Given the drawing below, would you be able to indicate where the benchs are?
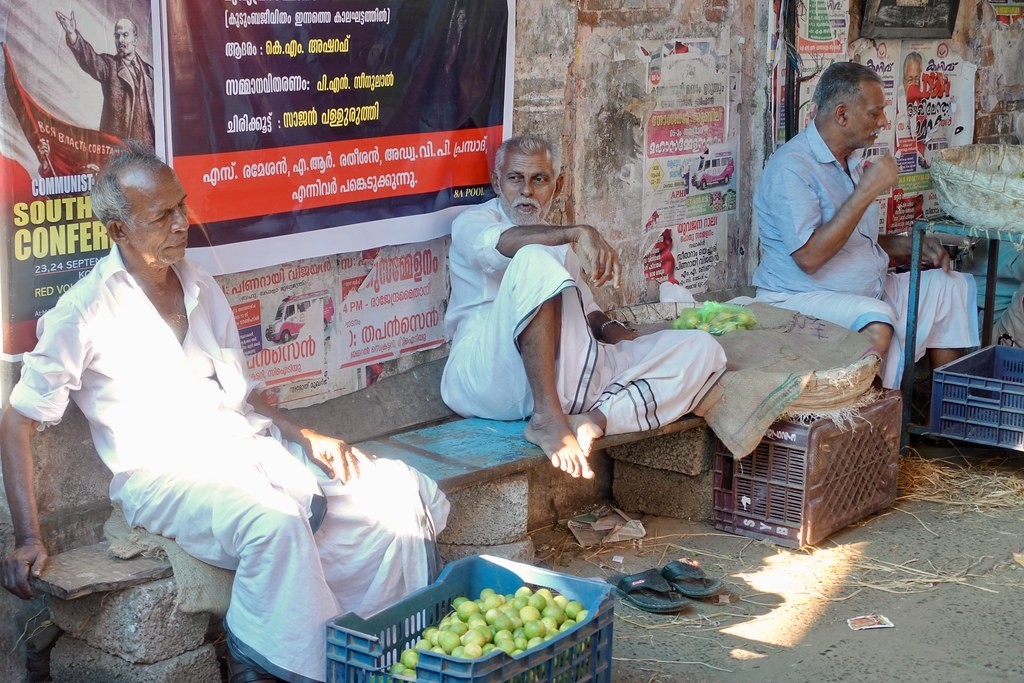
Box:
[21,417,713,683]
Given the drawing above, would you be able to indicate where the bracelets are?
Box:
[601,319,627,336]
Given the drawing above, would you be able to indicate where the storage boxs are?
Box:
[711,395,903,549]
[929,343,1024,452]
[325,554,618,683]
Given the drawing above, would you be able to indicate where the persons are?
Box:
[896,51,941,144]
[0,139,450,683]
[440,135,727,479]
[746,62,982,447]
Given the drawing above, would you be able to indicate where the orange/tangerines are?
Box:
[368,587,591,683]
[673,304,757,335]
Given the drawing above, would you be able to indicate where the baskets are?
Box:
[324,554,613,683]
[713,389,903,552]
[927,343,1024,453]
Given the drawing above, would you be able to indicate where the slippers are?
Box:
[661,561,725,599]
[604,568,688,613]
